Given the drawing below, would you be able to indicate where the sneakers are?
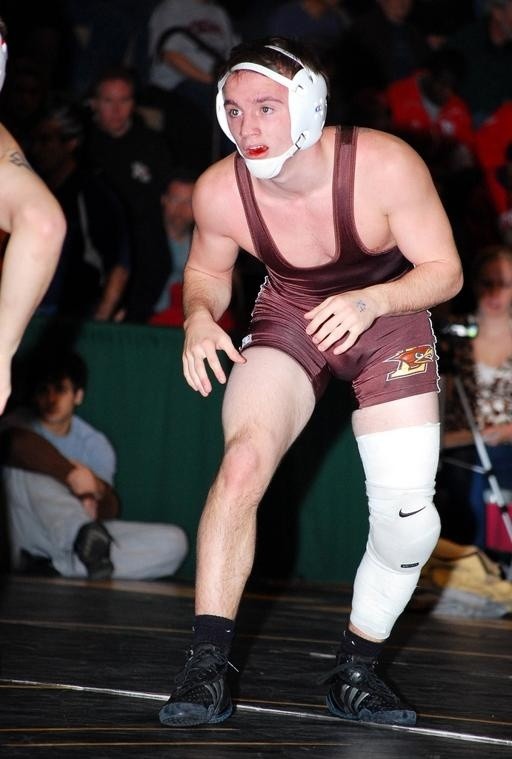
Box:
[74,521,120,580]
[160,642,240,726]
[317,649,417,725]
